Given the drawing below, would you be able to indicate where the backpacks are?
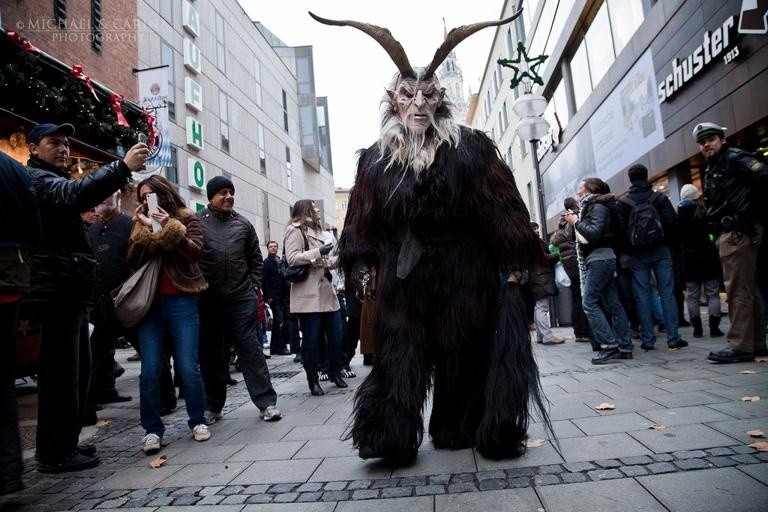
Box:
[627,203,667,249]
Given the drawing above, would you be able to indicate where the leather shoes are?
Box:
[263,347,292,359]
[35,441,101,473]
[306,374,324,396]
[590,350,633,366]
[754,346,768,356]
[707,345,754,362]
[327,374,349,388]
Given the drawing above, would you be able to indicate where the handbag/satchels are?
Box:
[108,259,165,330]
[278,257,310,283]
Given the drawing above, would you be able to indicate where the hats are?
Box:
[24,120,78,145]
[206,176,237,200]
[679,184,702,201]
[691,120,725,143]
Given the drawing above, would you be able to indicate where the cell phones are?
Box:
[138,134,146,148]
[146,193,162,232]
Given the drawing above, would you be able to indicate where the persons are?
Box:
[125,175,212,452]
[260,200,360,395]
[18,122,151,474]
[692,122,768,363]
[529,164,725,364]
[0,146,47,497]
[336,68,554,459]
[79,160,245,405]
[185,175,283,426]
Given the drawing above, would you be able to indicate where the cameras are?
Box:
[567,209,574,216]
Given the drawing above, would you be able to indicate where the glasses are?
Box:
[139,187,158,206]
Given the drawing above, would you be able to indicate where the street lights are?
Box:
[497,41,561,329]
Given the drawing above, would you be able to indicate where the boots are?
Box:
[690,315,705,338]
[709,315,724,338]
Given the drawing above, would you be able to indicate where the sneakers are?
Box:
[140,431,164,456]
[341,369,356,379]
[536,335,565,344]
[227,375,238,387]
[658,319,690,332]
[257,403,282,422]
[192,423,212,442]
[125,353,141,361]
[202,409,225,426]
[318,371,329,382]
[667,338,689,351]
[574,335,592,342]
[639,341,655,351]
[111,393,133,405]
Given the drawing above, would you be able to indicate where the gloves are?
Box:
[319,241,335,255]
